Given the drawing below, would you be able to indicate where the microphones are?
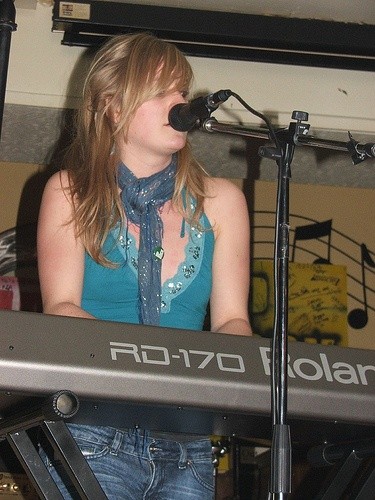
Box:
[168,90,231,132]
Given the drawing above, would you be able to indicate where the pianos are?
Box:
[0,309,375,425]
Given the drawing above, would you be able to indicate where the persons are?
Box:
[37,31,252,500]
